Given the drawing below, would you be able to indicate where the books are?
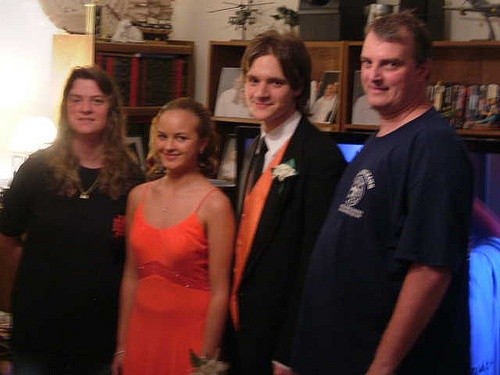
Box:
[98,56,189,107]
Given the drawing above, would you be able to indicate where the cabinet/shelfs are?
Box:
[205,40,500,214]
[52,35,195,174]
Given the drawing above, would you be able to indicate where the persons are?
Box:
[309,81,337,123]
[216,70,252,117]
[112,96,235,375]
[0,66,141,375]
[219,29,349,375]
[300,10,474,375]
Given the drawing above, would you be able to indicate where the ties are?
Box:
[251,135,269,188]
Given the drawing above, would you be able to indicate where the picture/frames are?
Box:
[310,70,341,124]
[211,65,259,120]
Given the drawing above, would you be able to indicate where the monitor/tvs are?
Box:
[235,126,376,190]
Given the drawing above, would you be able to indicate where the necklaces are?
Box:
[77,174,100,199]
